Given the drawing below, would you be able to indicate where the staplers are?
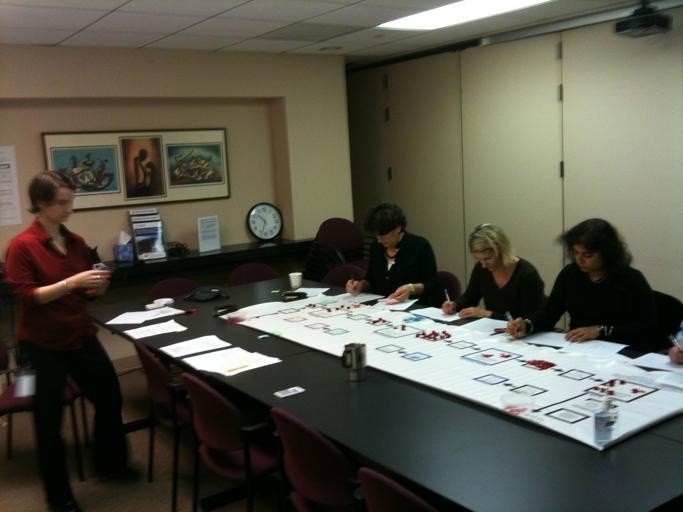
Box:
[282,292,307,302]
[214,304,238,317]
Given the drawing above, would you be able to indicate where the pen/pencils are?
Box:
[444,288,454,314]
[667,333,683,353]
[351,274,354,291]
[505,310,521,338]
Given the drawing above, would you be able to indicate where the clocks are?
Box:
[246,202,283,241]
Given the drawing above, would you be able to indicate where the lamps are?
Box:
[615,0,673,38]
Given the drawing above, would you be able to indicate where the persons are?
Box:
[668,319,683,364]
[3,170,140,512]
[505,218,661,351]
[442,223,546,319]
[345,202,439,301]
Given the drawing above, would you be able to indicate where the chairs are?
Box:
[228,263,281,288]
[1,339,83,472]
[84,272,683,512]
[322,265,367,289]
[307,218,362,269]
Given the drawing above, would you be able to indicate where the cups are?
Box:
[287,272,303,290]
[340,342,367,371]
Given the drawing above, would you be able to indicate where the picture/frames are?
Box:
[41,128,231,212]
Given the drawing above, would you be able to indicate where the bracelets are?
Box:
[598,319,617,340]
[409,283,416,296]
[525,318,534,335]
[62,278,71,295]
